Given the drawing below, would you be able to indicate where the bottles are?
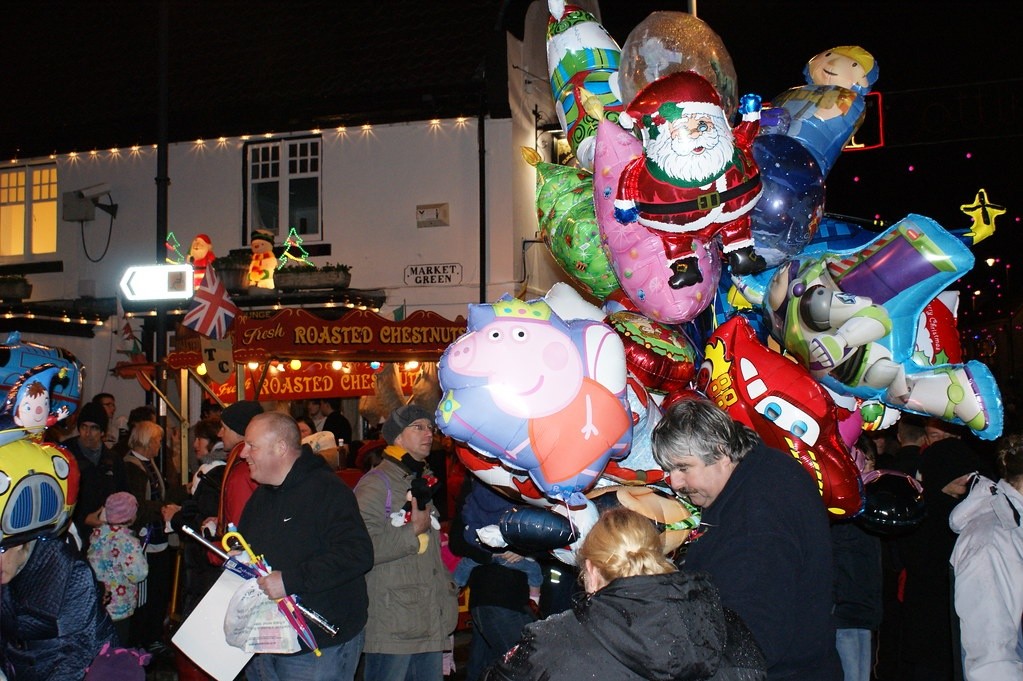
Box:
[336,439,348,470]
[228,522,242,548]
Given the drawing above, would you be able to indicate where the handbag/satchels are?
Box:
[84,642,152,681]
[244,596,302,653]
[224,577,265,651]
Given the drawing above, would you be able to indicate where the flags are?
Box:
[116,299,141,354]
[182,262,237,342]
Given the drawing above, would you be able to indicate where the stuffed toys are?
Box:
[388,475,441,556]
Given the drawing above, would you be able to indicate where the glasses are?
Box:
[406,425,433,432]
[103,402,115,407]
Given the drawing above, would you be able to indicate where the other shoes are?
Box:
[528,599,547,620]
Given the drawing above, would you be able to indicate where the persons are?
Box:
[87,492,149,653]
[827,413,1023,681]
[350,410,461,681]
[446,477,543,614]
[185,233,217,293]
[157,400,263,607]
[291,397,352,446]
[478,508,770,681]
[225,411,375,681]
[533,488,622,622]
[649,398,845,681]
[60,392,187,652]
[0,538,120,681]
[448,470,530,681]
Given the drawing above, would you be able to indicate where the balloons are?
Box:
[427,0,1006,552]
[12,367,70,445]
[0,425,81,546]
[0,330,84,426]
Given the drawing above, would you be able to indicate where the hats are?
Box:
[221,400,265,437]
[77,402,108,434]
[381,404,435,445]
[106,491,138,524]
[918,436,977,497]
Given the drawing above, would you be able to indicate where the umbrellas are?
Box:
[221,531,321,657]
